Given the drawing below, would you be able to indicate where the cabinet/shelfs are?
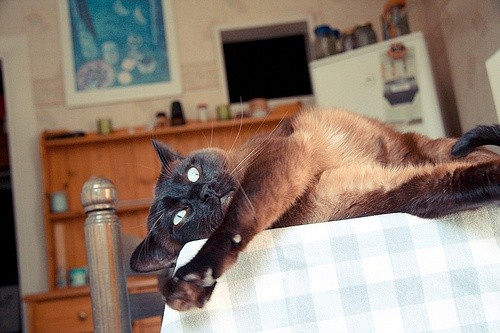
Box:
[23,101,302,333]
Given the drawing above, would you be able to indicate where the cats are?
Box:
[128,106,500,312]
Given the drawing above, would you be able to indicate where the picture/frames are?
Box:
[59,0,183,106]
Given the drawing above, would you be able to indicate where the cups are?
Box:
[217,105,228,120]
[97,119,111,135]
[70,268,88,288]
[51,190,67,213]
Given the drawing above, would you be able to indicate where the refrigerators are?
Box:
[308,31,446,139]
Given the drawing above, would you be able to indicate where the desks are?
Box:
[160,198,499,333]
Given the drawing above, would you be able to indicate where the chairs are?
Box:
[82,176,171,333]
